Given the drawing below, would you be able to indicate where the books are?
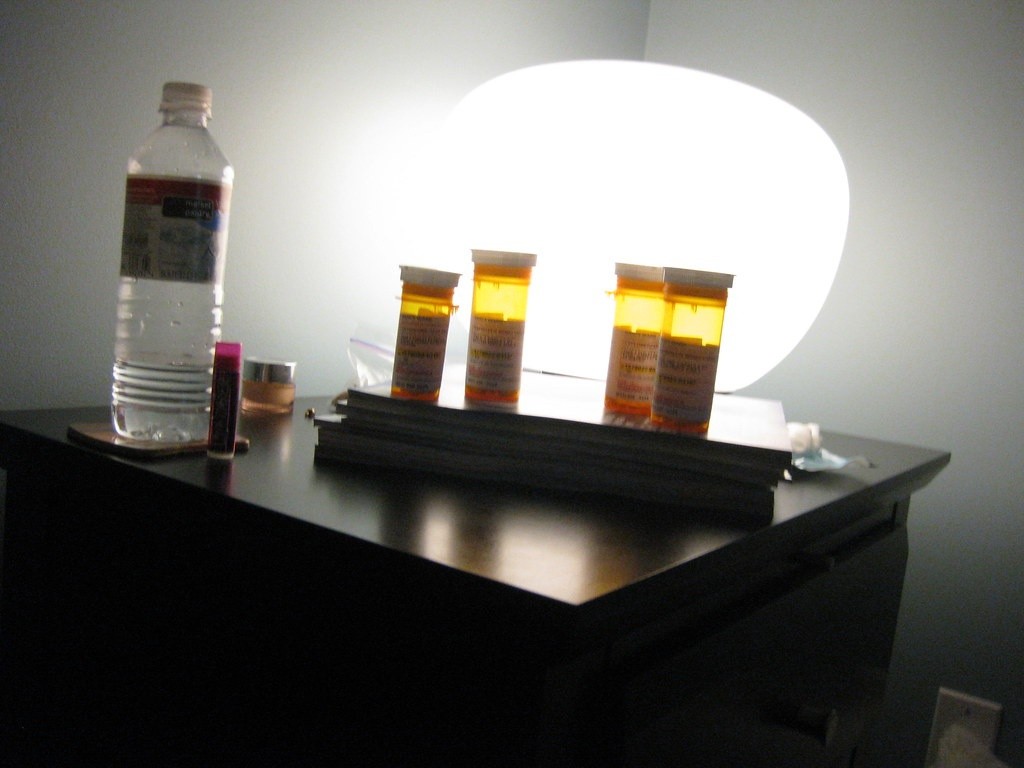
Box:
[312,362,792,516]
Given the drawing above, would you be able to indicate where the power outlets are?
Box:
[922,686,1002,768]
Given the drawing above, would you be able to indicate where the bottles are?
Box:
[393,266,462,400]
[112,82,234,440]
[605,263,666,416]
[652,267,737,432]
[465,249,538,408]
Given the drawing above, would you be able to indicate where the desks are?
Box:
[0,391,951,768]
